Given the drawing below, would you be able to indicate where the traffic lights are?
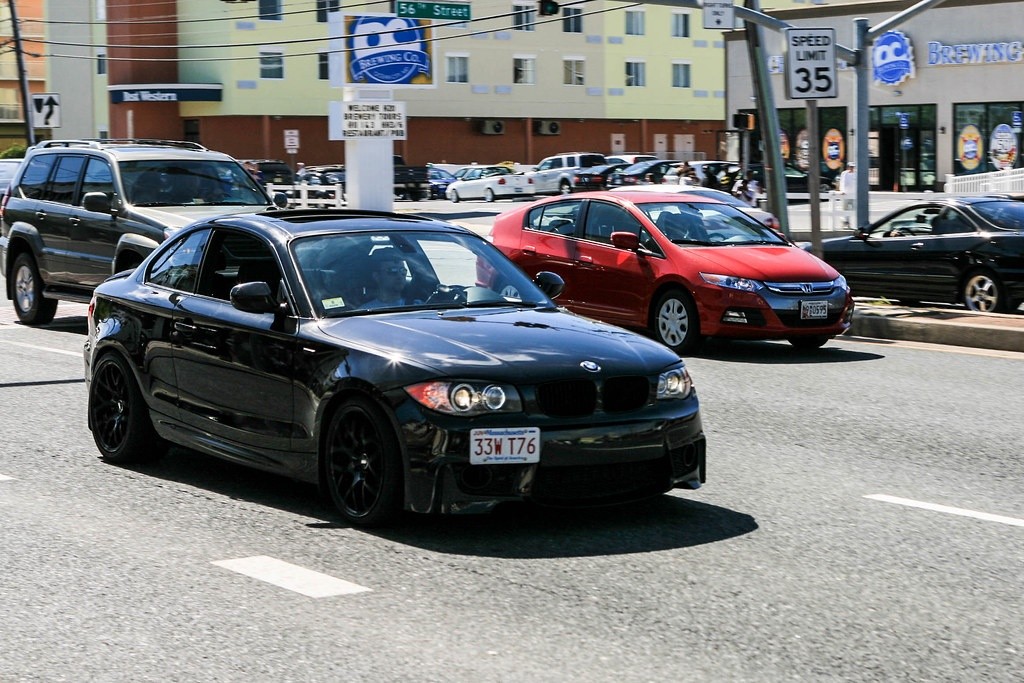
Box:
[537,0,559,17]
[732,113,755,130]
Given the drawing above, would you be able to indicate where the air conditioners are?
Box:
[538,121,561,134]
[480,120,506,135]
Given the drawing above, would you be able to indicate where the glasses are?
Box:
[379,267,407,276]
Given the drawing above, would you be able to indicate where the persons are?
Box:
[295,162,306,178]
[182,171,212,203]
[889,216,940,237]
[243,162,264,184]
[839,162,858,230]
[630,161,763,208]
[358,259,427,311]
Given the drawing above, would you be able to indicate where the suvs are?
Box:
[0,139,287,324]
[528,152,604,194]
[236,153,458,209]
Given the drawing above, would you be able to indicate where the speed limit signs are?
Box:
[784,26,838,99]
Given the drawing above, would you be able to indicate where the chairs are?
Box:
[938,220,966,232]
[657,209,672,236]
[593,205,621,237]
[237,242,282,296]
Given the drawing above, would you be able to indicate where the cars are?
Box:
[488,193,854,354]
[83,209,706,525]
[611,184,779,244]
[805,195,1024,312]
[456,167,474,177]
[445,167,535,203]
[575,155,832,193]
[0,158,26,194]
